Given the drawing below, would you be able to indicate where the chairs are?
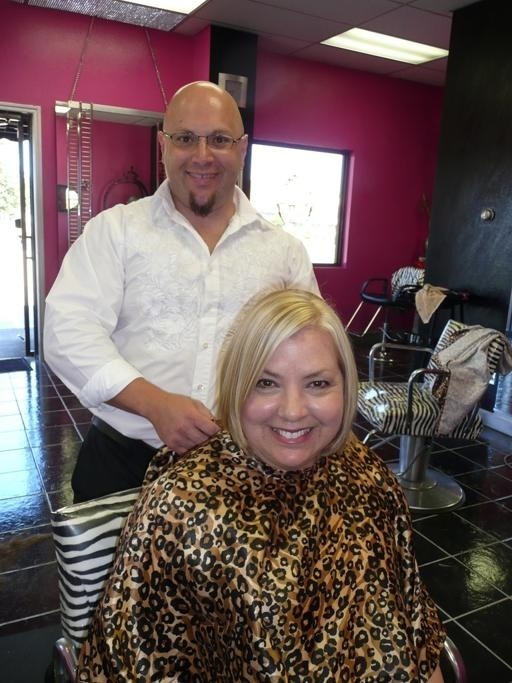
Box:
[354,320,505,512]
[50,485,466,683]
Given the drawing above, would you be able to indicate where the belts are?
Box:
[91,414,152,456]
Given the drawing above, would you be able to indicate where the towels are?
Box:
[415,284,449,324]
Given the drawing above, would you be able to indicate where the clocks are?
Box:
[218,72,248,109]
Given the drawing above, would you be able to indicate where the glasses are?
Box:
[163,133,243,150]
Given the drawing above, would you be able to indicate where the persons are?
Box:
[37,78,326,511]
[74,281,454,683]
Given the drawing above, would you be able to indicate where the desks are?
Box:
[406,289,467,348]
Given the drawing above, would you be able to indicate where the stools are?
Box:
[343,276,422,364]
